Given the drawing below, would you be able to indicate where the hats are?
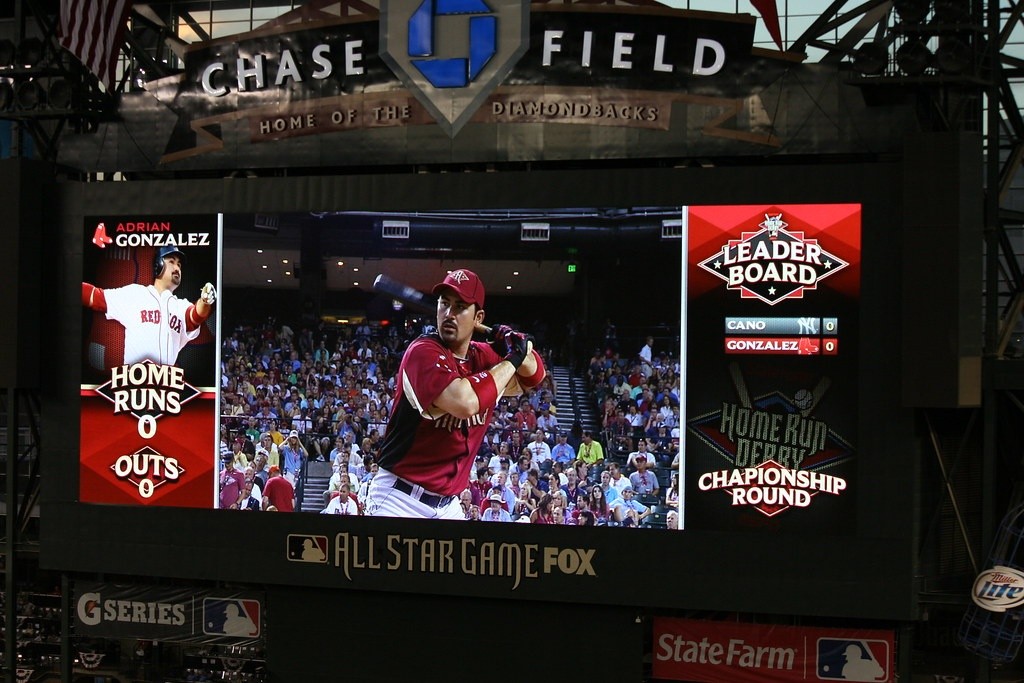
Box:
[221,451,235,462]
[431,269,485,309]
[267,465,280,479]
[258,449,270,456]
[152,244,186,268]
[636,455,648,462]
[559,430,568,437]
[475,456,484,463]
[540,403,550,411]
[498,458,510,464]
[231,437,244,446]
[289,430,300,439]
[487,494,507,504]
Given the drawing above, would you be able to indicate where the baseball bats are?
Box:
[371,273,498,337]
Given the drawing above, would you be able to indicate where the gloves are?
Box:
[484,324,536,370]
[200,282,217,306]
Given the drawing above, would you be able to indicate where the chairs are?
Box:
[219,323,680,530]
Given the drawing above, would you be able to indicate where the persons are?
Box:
[81,243,218,377]
[364,269,546,520]
[219,317,682,529]
